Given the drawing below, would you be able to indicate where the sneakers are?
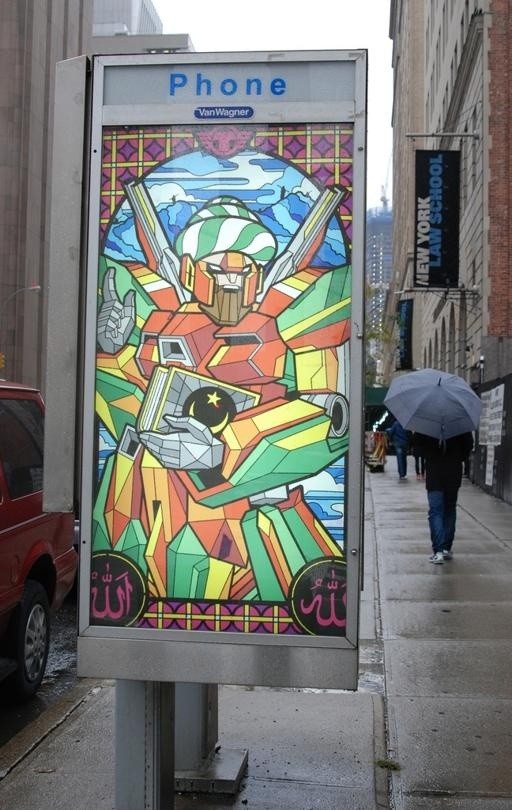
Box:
[400,475,408,480]
[429,550,453,564]
[416,475,426,480]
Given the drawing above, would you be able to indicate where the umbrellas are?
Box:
[382,366,486,446]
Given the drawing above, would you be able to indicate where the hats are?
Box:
[176,195,278,268]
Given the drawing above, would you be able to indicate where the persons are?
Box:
[384,425,393,455]
[410,430,427,479]
[389,419,413,480]
[417,432,474,565]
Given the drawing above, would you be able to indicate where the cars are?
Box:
[0,383,80,702]
